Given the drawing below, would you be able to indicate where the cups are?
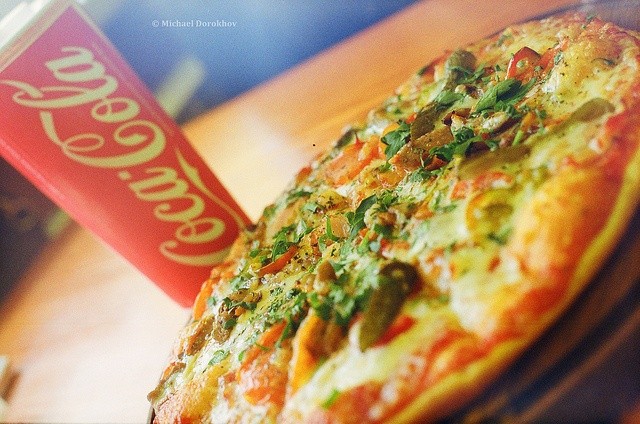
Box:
[1,0,256,314]
